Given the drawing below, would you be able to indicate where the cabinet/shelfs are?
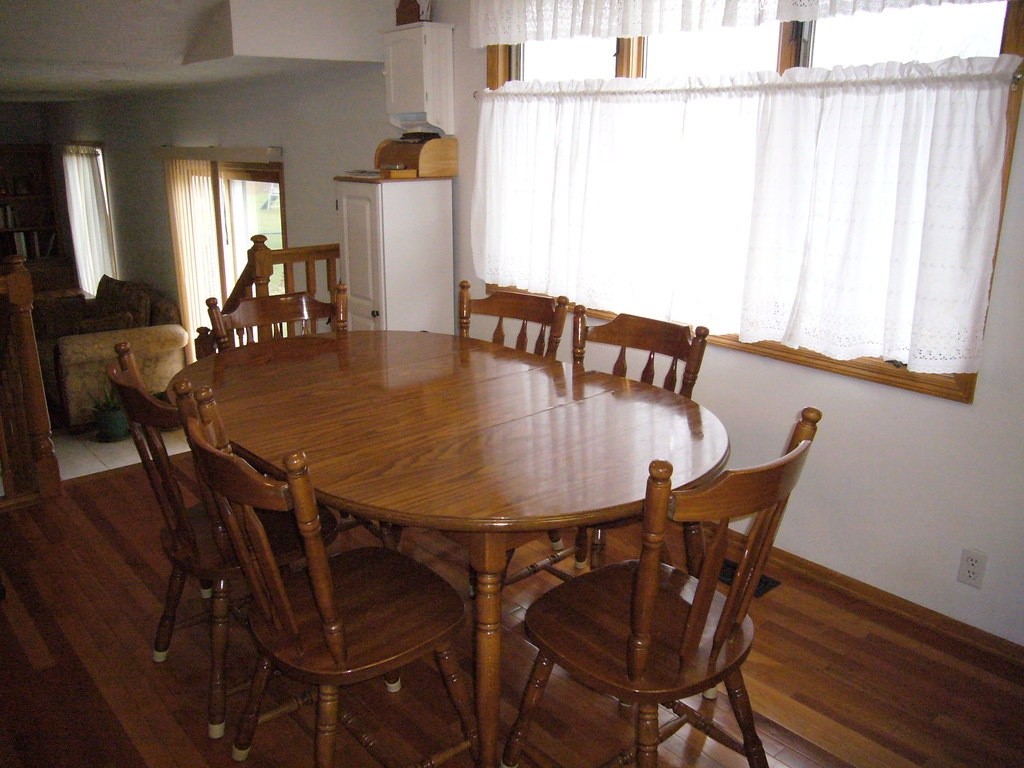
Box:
[0,144,76,289]
[382,22,455,136]
[334,172,453,334]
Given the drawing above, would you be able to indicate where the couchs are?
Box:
[32,273,189,434]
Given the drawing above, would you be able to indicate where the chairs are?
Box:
[501,407,823,768]
[184,385,480,768]
[105,342,340,740]
[206,282,401,553]
[501,305,710,590]
[391,281,569,599]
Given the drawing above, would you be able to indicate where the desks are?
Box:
[168,330,731,768]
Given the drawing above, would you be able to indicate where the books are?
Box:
[14,231,55,259]
[0,205,15,229]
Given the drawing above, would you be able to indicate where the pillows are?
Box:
[78,311,132,334]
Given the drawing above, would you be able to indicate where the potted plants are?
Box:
[87,384,130,442]
